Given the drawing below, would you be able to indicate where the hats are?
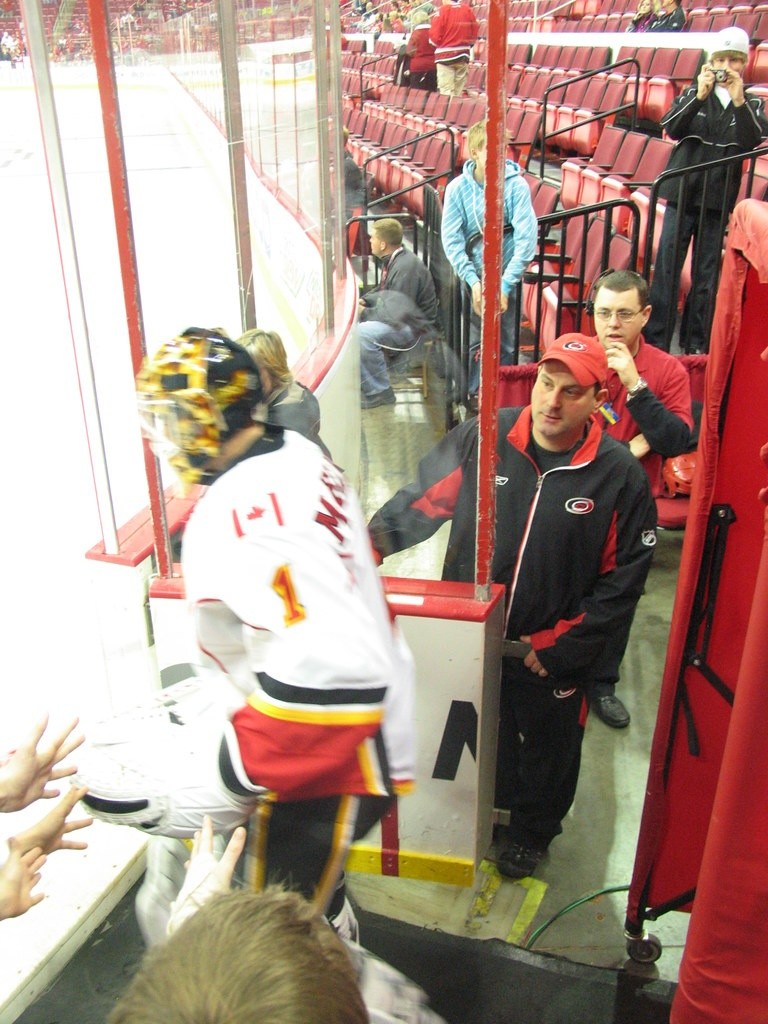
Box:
[708,26,749,56]
[536,333,610,398]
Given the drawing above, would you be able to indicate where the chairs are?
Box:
[0,0,312,63]
[325,0,768,529]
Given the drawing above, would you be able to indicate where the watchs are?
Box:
[629,377,647,395]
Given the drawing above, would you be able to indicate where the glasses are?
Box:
[594,305,648,323]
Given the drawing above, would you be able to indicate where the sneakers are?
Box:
[497,839,544,877]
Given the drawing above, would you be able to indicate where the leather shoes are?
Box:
[357,386,396,409]
[590,690,629,728]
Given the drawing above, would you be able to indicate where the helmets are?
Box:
[133,326,269,489]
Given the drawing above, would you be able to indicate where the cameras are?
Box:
[708,69,727,83]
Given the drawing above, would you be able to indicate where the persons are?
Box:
[643,0,686,33]
[440,122,538,398]
[0,326,415,1024]
[626,0,664,32]
[360,219,437,408]
[645,27,768,353]
[0,0,218,65]
[238,329,332,464]
[584,269,693,727]
[406,12,437,92]
[344,127,363,206]
[355,0,434,31]
[366,331,658,881]
[430,0,480,96]
